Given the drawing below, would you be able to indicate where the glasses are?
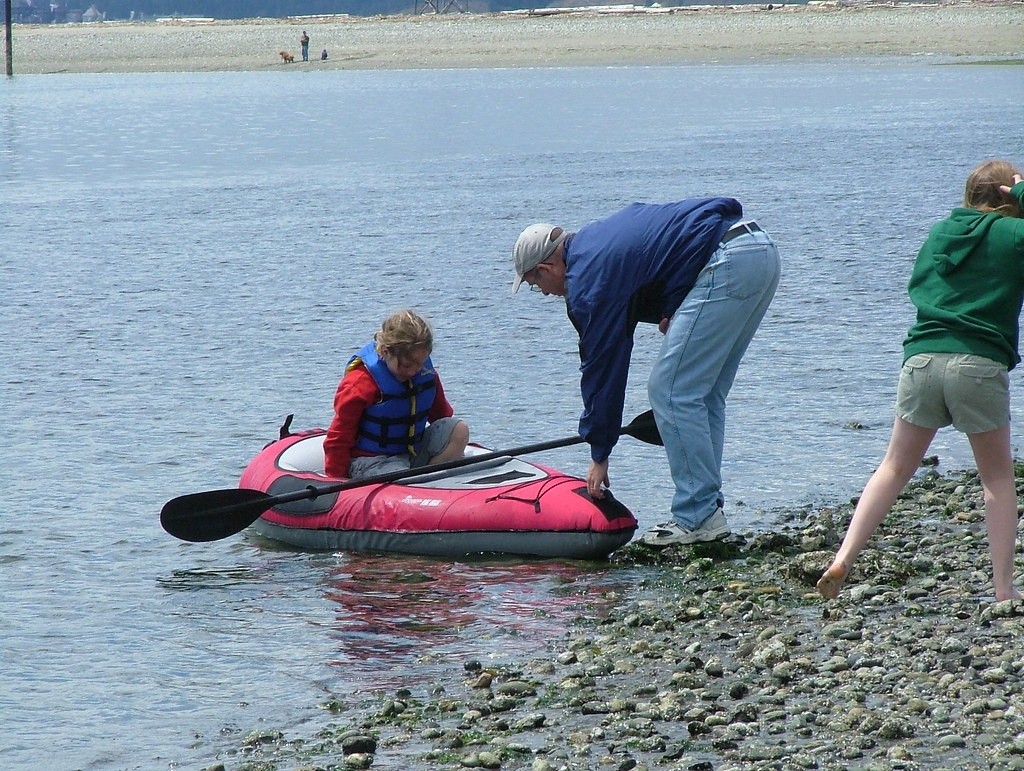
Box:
[531,270,542,292]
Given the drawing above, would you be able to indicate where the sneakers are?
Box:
[640,507,731,545]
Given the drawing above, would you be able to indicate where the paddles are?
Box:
[159,405,665,544]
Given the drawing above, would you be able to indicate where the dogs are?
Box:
[279,51,293,63]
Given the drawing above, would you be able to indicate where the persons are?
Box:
[300,31,309,61]
[322,310,469,480]
[511,199,781,545]
[815,160,1024,599]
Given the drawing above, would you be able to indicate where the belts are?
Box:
[714,221,761,250]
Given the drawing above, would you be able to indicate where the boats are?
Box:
[236,414,640,562]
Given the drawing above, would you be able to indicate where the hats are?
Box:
[512,223,567,294]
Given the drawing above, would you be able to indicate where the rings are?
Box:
[590,487,594,490]
